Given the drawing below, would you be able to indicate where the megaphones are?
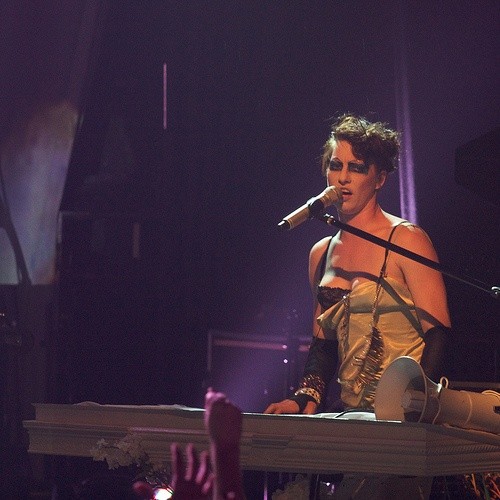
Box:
[374,355,500,437]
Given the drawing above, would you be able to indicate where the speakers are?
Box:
[207,330,290,413]
[52,210,186,404]
[289,335,343,413]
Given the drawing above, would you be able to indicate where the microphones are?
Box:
[277,185,342,232]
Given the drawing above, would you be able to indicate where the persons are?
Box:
[128,383,248,500]
[252,111,459,500]
